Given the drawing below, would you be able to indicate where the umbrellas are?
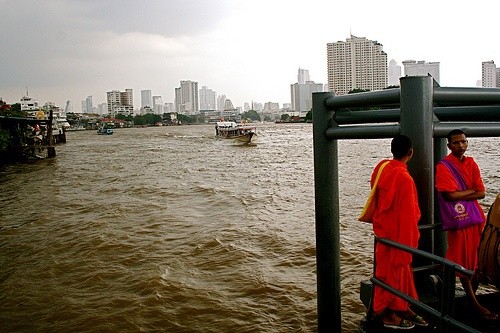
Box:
[0,104,12,109]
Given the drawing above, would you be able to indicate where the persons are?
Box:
[435,129,498,323]
[370,134,428,330]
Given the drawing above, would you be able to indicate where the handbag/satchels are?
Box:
[357,161,391,223]
[435,159,483,231]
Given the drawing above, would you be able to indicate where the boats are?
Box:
[20,88,86,144]
[215,117,258,147]
[97,127,114,135]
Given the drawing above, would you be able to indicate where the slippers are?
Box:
[406,314,430,326]
[384,317,415,329]
